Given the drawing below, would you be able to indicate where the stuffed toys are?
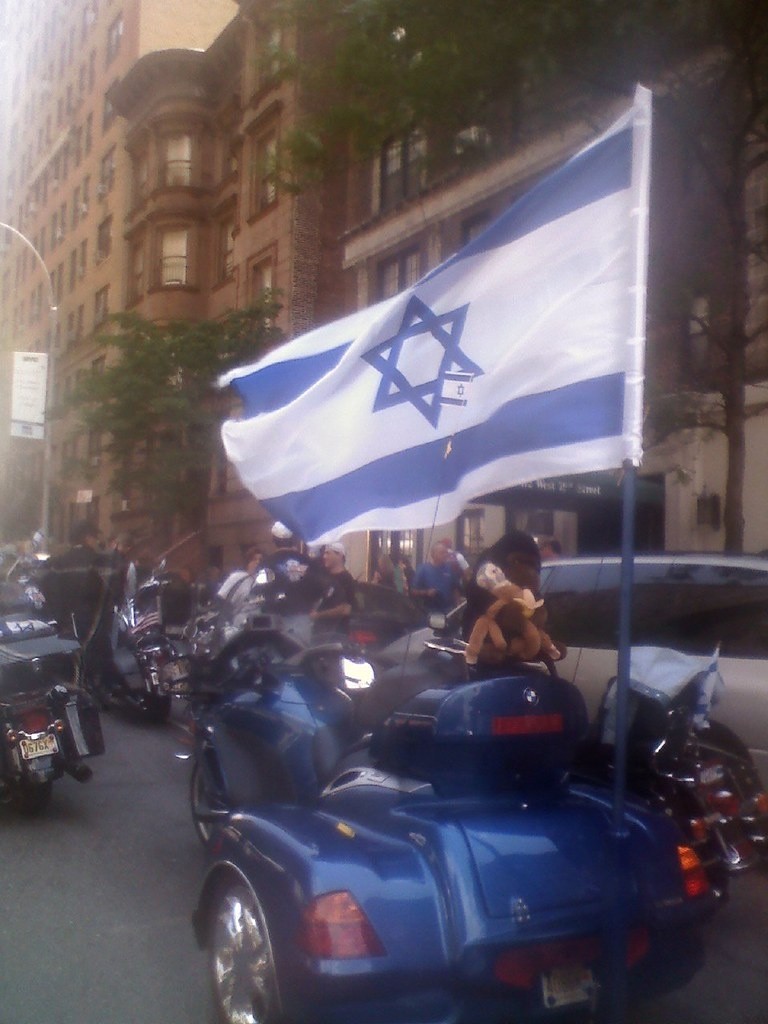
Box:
[461,529,568,678]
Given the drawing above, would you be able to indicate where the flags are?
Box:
[211,81,656,555]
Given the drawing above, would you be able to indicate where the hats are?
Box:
[271,521,293,545]
[326,542,346,557]
[69,518,103,536]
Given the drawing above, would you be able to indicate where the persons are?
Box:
[307,543,359,643]
[202,519,323,639]
[372,538,471,620]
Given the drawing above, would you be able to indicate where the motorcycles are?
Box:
[7,531,768,925]
[3,574,106,810]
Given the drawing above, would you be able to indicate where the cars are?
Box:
[367,553,766,772]
[173,642,730,1022]
